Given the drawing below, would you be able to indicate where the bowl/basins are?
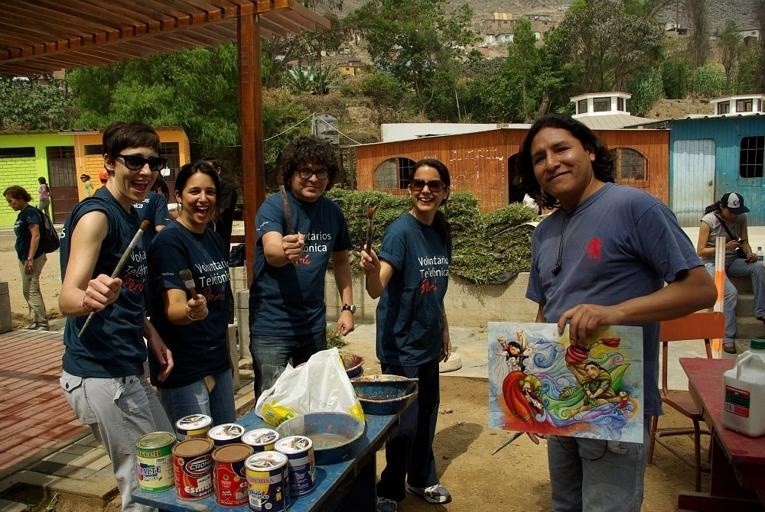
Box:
[275,411,364,466]
[348,374,418,416]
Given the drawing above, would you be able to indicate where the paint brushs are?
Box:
[76,220,147,338]
[365,206,379,257]
[277,173,295,237]
[492,432,526,456]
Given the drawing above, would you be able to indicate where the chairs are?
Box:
[648,308,728,493]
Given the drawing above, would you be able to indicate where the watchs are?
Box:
[338,304,358,314]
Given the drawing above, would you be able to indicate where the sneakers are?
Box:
[376,496,398,511]
[406,482,451,504]
[724,342,735,353]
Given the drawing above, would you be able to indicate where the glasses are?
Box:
[297,168,331,180]
[119,154,168,171]
[410,178,447,192]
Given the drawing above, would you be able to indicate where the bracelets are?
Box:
[23,255,32,259]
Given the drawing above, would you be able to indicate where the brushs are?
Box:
[179,269,200,301]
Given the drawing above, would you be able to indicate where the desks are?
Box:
[123,399,410,511]
[678,352,764,512]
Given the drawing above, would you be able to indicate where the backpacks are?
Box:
[37,207,60,253]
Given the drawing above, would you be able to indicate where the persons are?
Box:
[359,157,454,512]
[128,190,166,239]
[78,173,93,199]
[36,177,53,220]
[57,122,177,512]
[515,113,717,509]
[696,192,765,353]
[243,133,358,406]
[87,168,107,197]
[204,161,237,264]
[144,160,237,434]
[2,185,60,334]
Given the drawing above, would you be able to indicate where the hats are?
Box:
[721,192,750,214]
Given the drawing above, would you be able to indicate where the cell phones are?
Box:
[738,240,744,243]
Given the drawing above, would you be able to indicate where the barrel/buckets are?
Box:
[722,336,764,437]
